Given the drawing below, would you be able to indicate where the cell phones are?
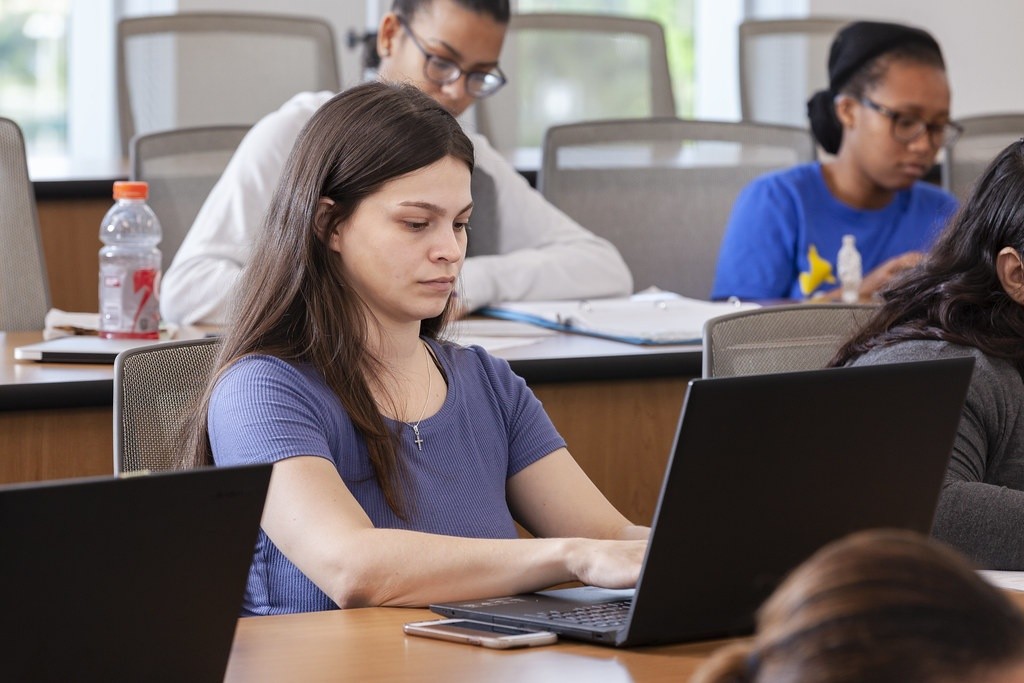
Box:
[403,618,557,650]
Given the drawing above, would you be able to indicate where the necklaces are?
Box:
[375,340,433,450]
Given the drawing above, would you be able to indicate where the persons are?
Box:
[708,19,962,308]
[166,81,651,623]
[824,140,1023,573]
[685,523,1023,682]
[158,1,632,325]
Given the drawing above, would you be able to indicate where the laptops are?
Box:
[428,355,979,651]
[0,461,276,683]
[14,336,166,363]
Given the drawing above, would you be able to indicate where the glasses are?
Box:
[854,89,964,149]
[394,16,507,99]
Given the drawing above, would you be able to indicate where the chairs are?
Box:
[0,0,1023,475]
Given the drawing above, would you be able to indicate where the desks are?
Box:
[222,556,1024,683]
[0,303,797,528]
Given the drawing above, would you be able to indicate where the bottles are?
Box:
[99,181,162,342]
[837,235,862,304]
